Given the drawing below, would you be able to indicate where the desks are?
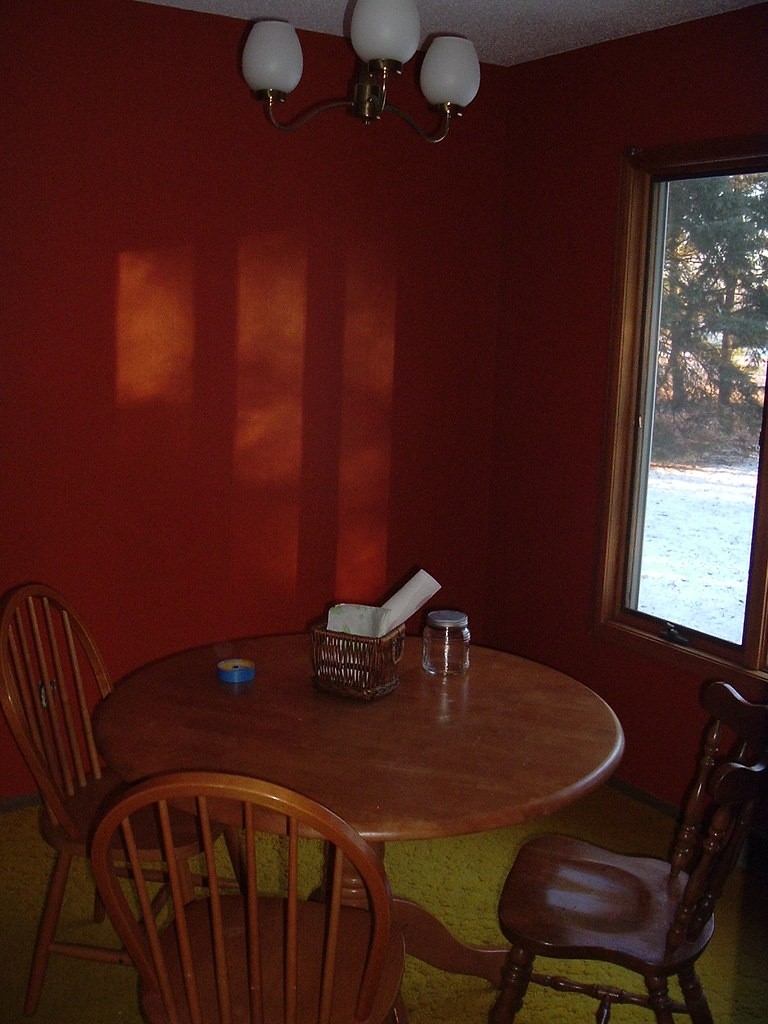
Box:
[94,633,624,1023]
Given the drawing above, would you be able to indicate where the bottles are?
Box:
[420,611,471,674]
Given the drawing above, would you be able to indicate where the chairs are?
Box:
[488,681,768,1024]
[91,771,406,1024]
[0,582,246,1016]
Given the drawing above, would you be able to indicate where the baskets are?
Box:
[310,621,407,700]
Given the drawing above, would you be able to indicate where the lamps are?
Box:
[242,1,481,146]
